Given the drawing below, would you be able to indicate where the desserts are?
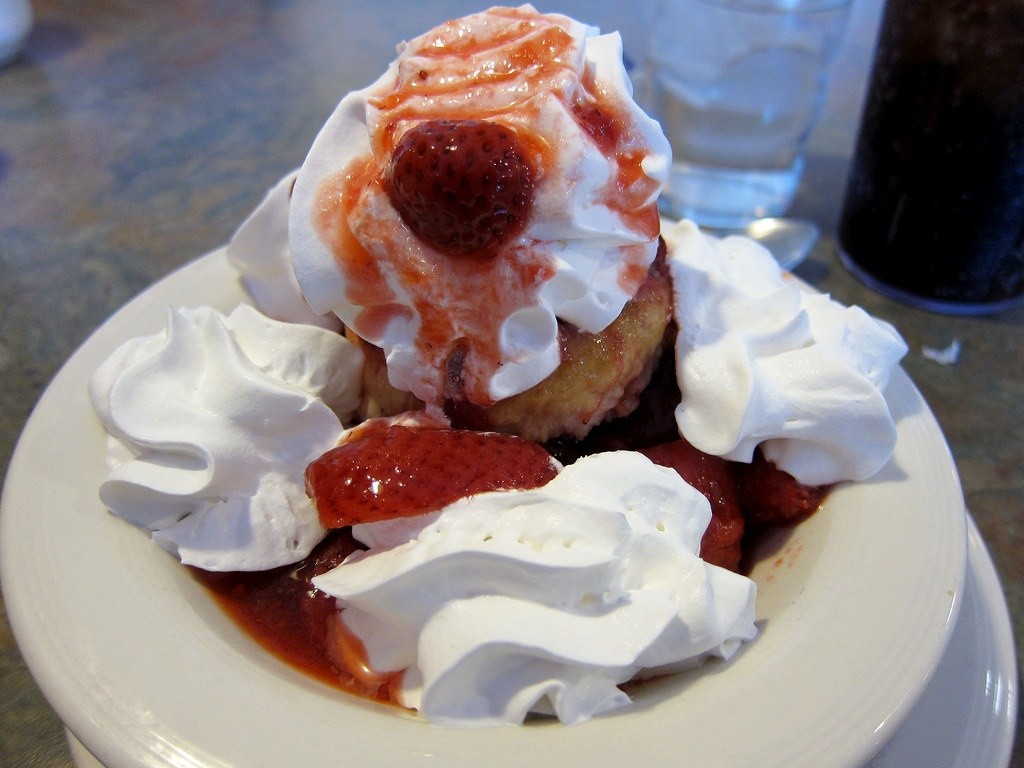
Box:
[86,0,915,728]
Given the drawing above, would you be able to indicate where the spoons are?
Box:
[743,218,821,272]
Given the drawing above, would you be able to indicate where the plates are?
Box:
[64,510,1017,767]
[1,243,967,767]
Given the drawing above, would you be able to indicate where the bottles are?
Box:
[835,0,1024,317]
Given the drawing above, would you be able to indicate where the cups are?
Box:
[629,1,854,231]
